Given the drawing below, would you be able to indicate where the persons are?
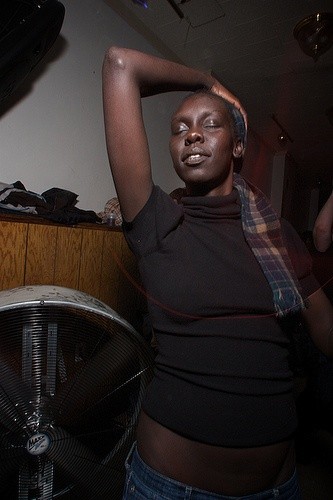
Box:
[312,190,333,255]
[102,45,332,500]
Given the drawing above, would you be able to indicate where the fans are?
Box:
[1,285,148,500]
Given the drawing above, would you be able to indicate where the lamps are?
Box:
[272,116,294,145]
[294,12,333,61]
